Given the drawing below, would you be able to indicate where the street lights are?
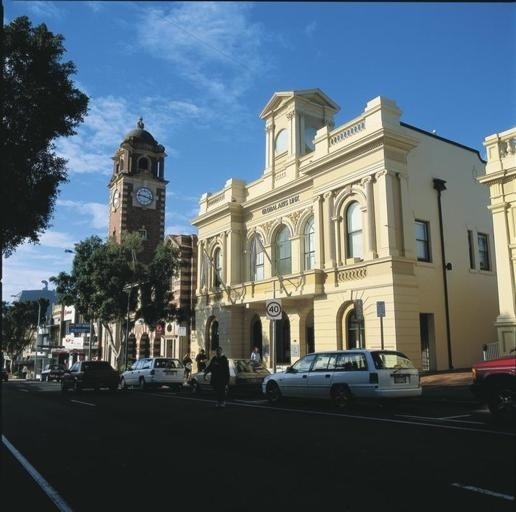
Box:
[64,248,76,254]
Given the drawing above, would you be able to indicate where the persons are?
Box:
[182,350,208,378]
[251,347,260,363]
[203,347,229,408]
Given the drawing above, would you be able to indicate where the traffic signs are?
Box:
[266,299,282,320]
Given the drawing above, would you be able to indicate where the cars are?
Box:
[1,367,8,382]
[472,348,516,414]
